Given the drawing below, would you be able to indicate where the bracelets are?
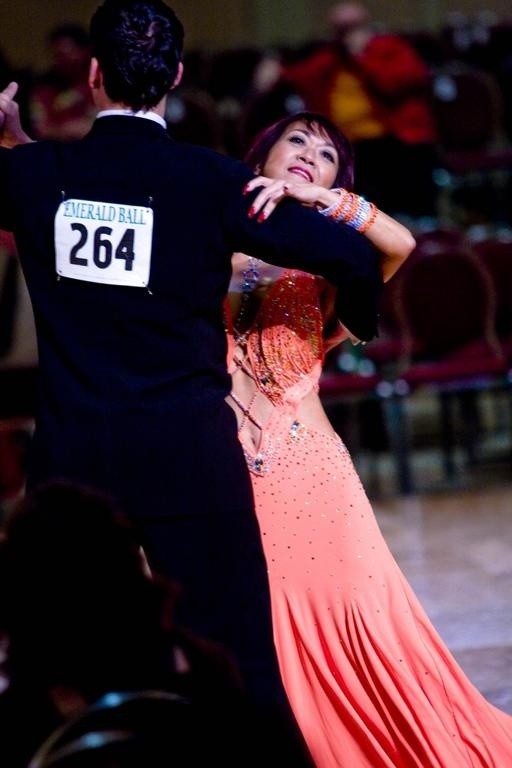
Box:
[316,184,379,235]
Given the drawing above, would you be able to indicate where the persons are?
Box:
[0,1,386,767]
[0,1,512,237]
[0,77,512,767]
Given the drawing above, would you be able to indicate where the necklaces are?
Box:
[234,251,268,328]
